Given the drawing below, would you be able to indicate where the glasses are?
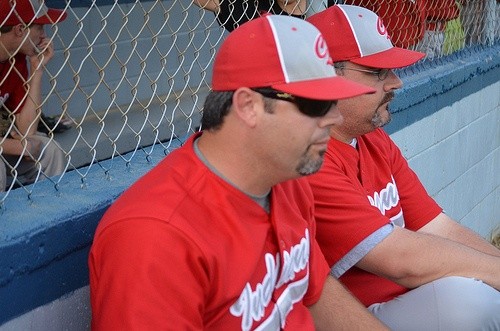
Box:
[229,87,338,117]
[335,66,389,80]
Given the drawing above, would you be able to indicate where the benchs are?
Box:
[20,0,239,169]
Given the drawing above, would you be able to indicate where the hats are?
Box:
[212,13,376,100]
[305,5,426,69]
[0,0,67,26]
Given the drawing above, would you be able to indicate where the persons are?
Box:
[88,12,391,331]
[193,0,307,33]
[305,4,500,331]
[0,0,67,191]
